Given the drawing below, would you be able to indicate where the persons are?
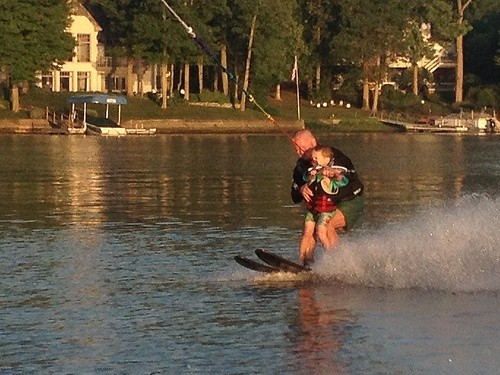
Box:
[298,146,349,267]
[291,127,364,269]
